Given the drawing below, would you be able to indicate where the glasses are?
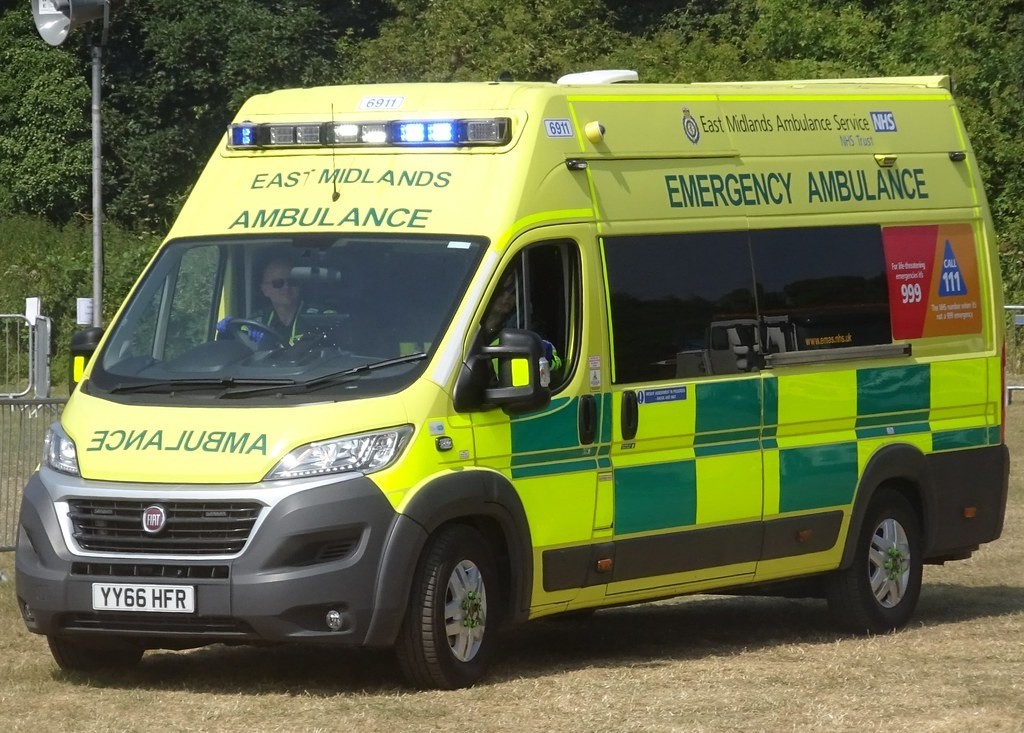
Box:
[265,277,301,288]
[498,284,516,297]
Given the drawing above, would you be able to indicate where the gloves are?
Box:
[216,316,233,338]
[249,323,273,343]
[542,339,553,366]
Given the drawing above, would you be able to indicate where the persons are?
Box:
[216,260,338,346]
[401,269,561,382]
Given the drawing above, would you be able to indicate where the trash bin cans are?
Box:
[68,328,105,397]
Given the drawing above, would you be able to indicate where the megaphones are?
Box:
[32,0,105,45]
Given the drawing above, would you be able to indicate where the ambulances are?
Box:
[13,69,1012,693]
